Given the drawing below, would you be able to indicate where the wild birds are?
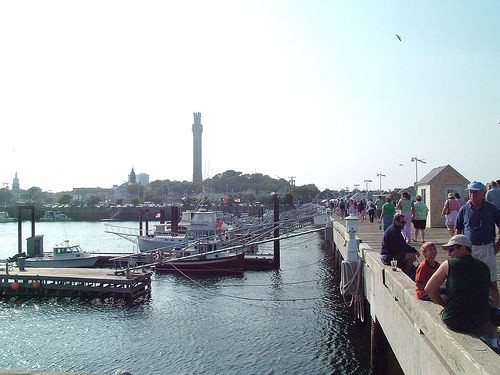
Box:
[393,32,406,44]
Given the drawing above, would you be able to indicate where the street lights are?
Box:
[377,173,386,194]
[411,156,427,182]
[364,179,373,193]
[353,184,360,192]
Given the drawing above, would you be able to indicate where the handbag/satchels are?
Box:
[370,203,376,208]
[444,200,450,214]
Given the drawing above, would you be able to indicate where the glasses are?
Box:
[447,248,454,253]
[399,221,406,224]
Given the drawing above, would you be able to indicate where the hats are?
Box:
[465,181,487,192]
[441,234,472,251]
[448,193,454,198]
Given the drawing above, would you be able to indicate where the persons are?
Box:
[326,199,375,223]
[454,181,500,309]
[373,196,384,219]
[438,180,500,237]
[391,199,400,207]
[380,214,420,277]
[411,195,429,242]
[397,192,415,244]
[424,235,500,353]
[415,242,446,300]
[378,197,395,232]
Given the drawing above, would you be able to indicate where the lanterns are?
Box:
[11,282,19,290]
[32,281,40,288]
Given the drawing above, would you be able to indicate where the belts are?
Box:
[474,242,491,246]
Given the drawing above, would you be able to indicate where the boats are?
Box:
[39,210,72,221]
[136,204,261,272]
[5,240,100,269]
[0,211,18,223]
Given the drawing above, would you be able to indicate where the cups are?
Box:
[391,261,397,271]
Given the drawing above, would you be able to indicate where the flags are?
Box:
[223,198,228,202]
[234,198,240,202]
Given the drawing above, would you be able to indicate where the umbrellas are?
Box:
[320,193,366,204]
